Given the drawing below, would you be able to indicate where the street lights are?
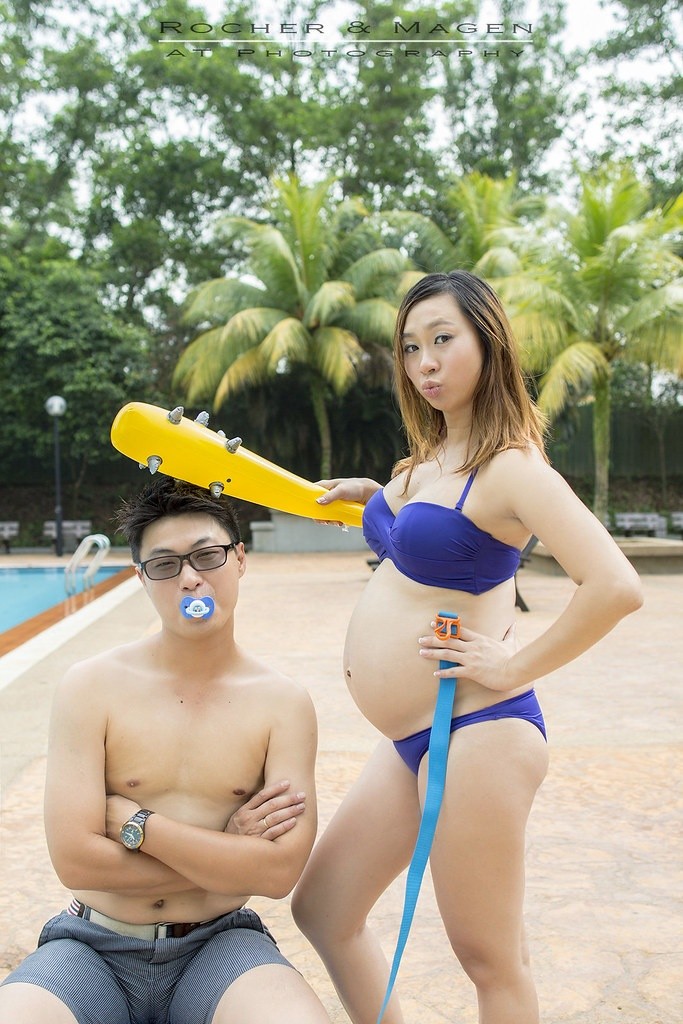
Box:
[44,394,68,557]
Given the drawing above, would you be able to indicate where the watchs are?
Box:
[119,808,155,853]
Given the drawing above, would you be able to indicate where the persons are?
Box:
[2,470,341,1024]
[290,272,641,1024]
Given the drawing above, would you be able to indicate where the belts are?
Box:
[67,897,245,941]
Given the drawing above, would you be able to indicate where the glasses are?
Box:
[137,541,239,581]
